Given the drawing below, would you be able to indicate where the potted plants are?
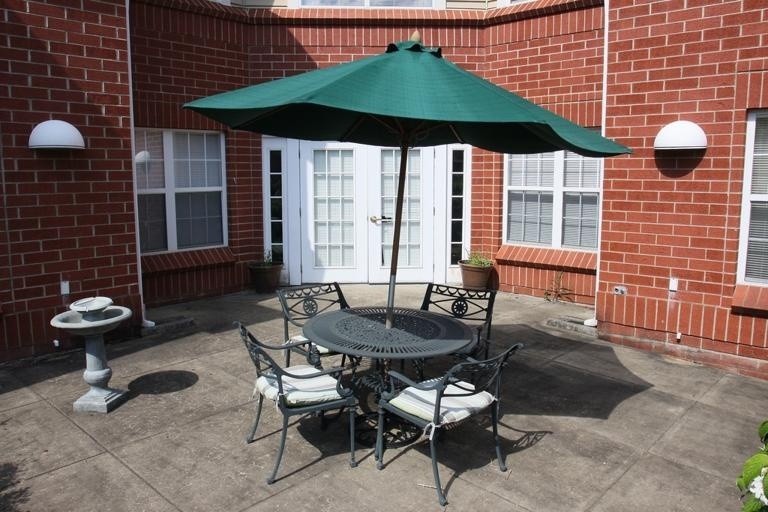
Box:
[457,242,497,293]
[247,246,286,295]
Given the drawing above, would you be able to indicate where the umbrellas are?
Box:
[180,30,634,330]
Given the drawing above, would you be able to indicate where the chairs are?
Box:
[307,305,473,449]
[375,343,523,508]
[399,282,496,386]
[277,283,364,427]
[234,321,357,484]
